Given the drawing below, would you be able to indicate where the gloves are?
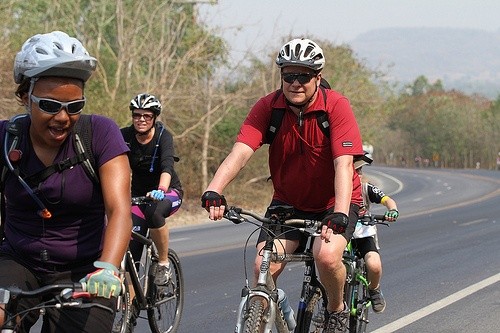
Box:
[146,189,164,201]
[79,259,123,299]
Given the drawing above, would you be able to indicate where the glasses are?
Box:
[132,112,154,121]
[27,92,86,114]
[281,72,317,84]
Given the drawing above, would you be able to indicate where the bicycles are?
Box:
[109,192,186,333]
[341,209,398,333]
[0,279,126,333]
[201,193,351,333]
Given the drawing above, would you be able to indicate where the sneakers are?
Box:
[111,317,134,333]
[154,261,171,286]
[322,298,350,333]
[368,282,386,314]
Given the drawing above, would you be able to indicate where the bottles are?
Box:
[276,288,297,330]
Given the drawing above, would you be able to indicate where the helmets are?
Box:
[13,31,98,83]
[276,38,326,75]
[353,151,373,169]
[129,94,161,116]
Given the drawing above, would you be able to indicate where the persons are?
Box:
[201,38,365,333]
[119,93,184,326]
[346,151,398,313]
[0,31,132,333]
